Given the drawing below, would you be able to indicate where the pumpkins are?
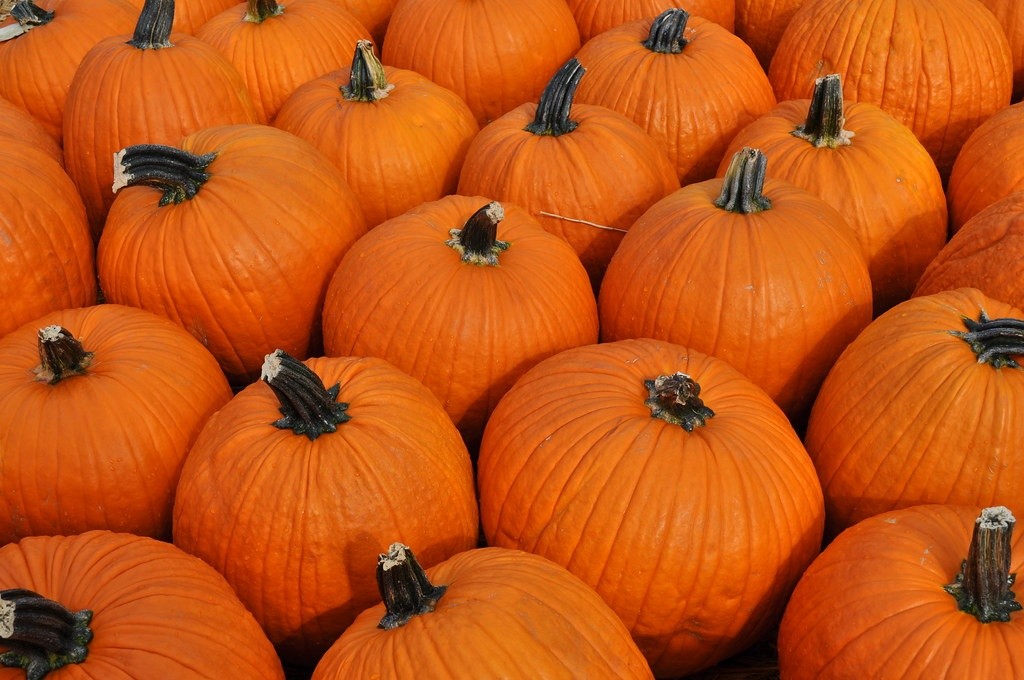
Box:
[0,0,1024,680]
